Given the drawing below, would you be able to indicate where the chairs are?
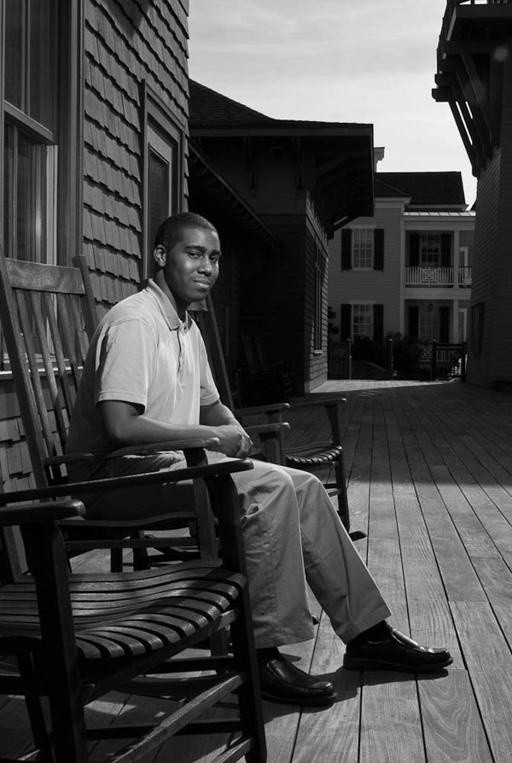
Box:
[0,255,291,699]
[145,273,366,546]
[1,457,271,763]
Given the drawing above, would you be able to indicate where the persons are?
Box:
[328,326,342,360]
[72,211,453,706]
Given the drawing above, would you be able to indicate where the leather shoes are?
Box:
[259,654,339,707]
[343,626,453,674]
